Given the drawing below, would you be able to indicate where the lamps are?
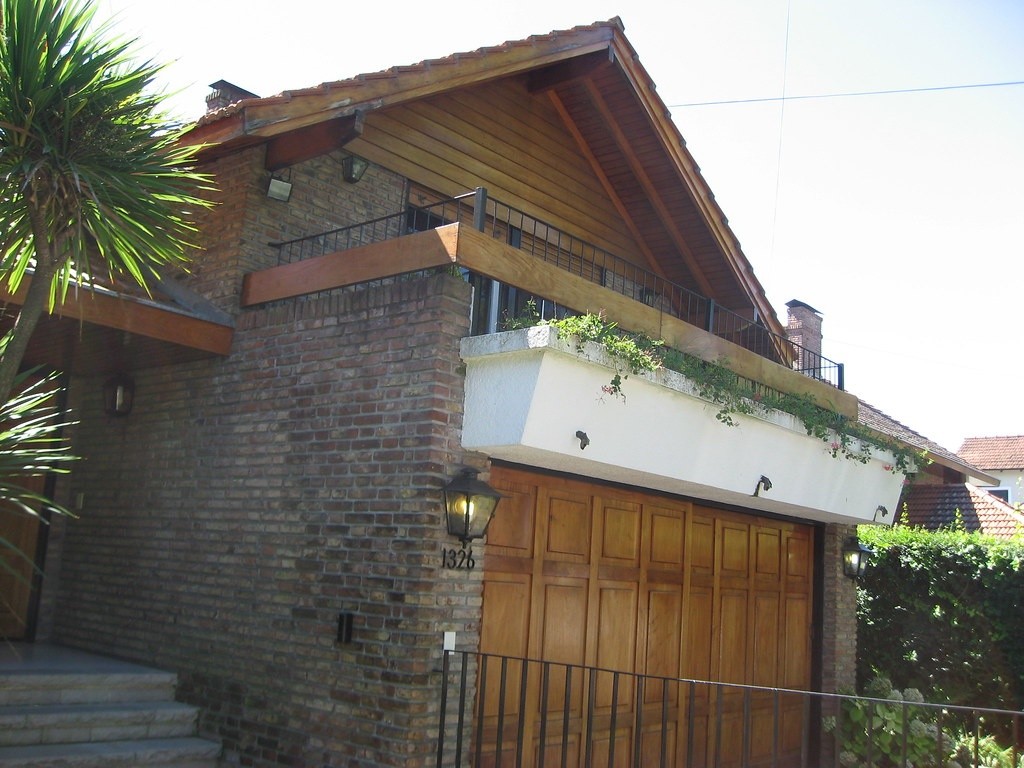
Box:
[337,612,353,645]
[266,166,294,203]
[574,430,590,450]
[441,467,512,550]
[341,154,371,185]
[752,473,773,497]
[873,506,889,524]
[840,536,874,579]
[104,371,135,418]
